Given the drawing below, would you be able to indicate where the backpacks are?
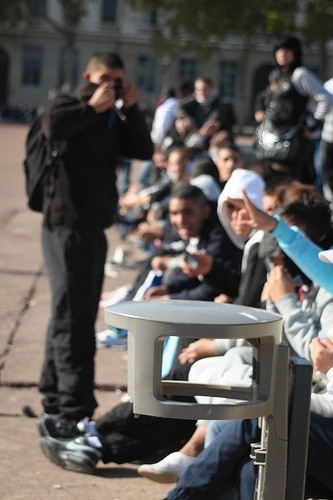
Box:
[25,114,58,211]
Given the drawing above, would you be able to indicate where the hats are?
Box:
[319,247,333,264]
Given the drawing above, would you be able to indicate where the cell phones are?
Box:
[183,252,199,270]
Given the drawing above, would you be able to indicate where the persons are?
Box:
[36,51,153,419]
[40,35,333,500]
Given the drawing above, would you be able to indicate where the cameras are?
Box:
[110,82,126,100]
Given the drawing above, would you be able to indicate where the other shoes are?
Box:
[39,438,103,473]
[139,451,197,484]
[94,328,128,349]
[40,417,90,440]
